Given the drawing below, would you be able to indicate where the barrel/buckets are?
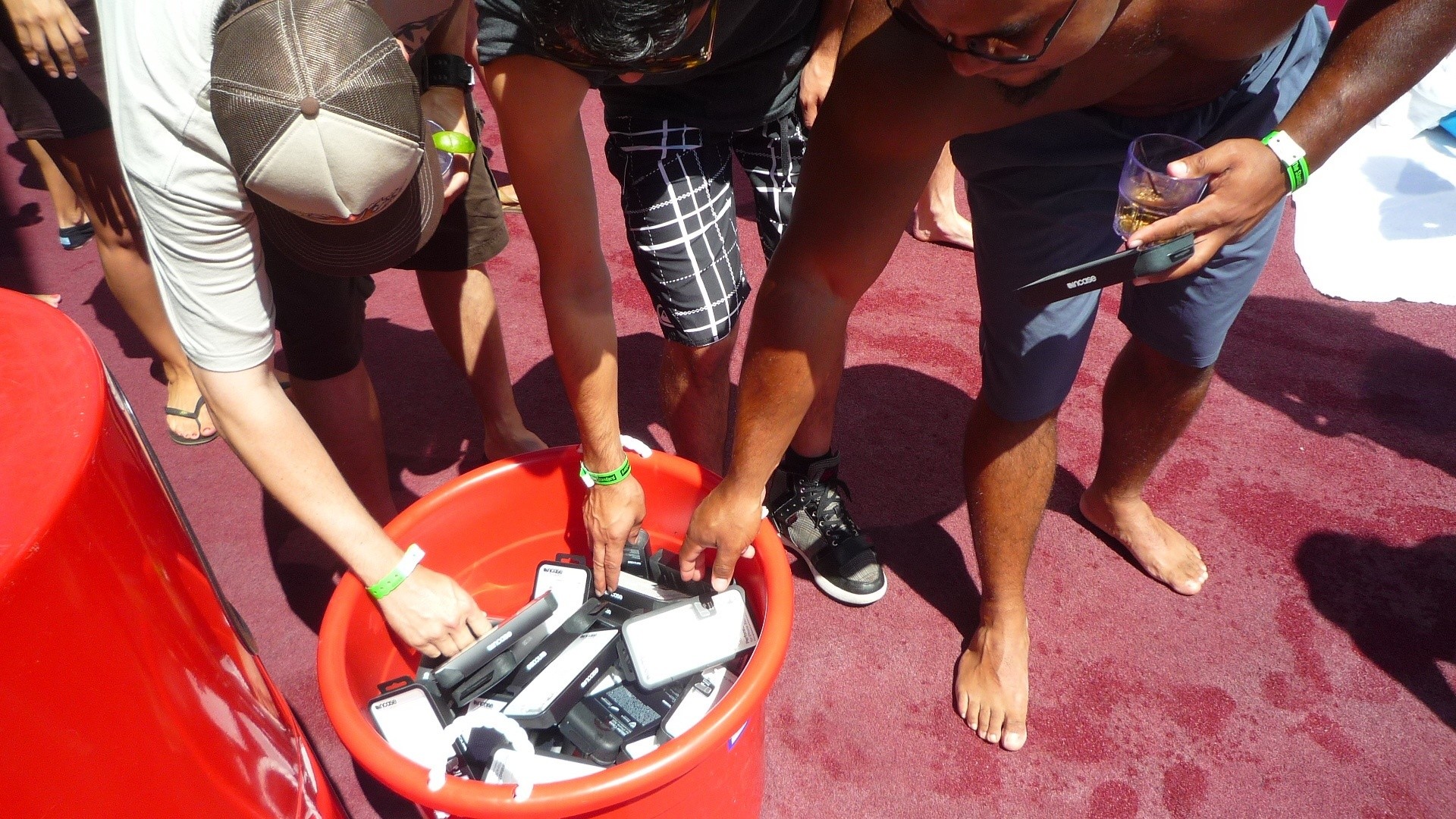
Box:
[315,446,796,819]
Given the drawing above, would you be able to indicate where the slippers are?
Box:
[164,395,218,446]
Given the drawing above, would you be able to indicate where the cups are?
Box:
[1111,132,1212,244]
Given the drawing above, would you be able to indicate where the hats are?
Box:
[209,0,444,279]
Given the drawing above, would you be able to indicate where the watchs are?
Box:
[411,49,475,97]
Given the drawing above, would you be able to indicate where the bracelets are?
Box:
[578,452,632,490]
[1259,128,1309,192]
[364,543,425,600]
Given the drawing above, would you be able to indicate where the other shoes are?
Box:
[59,220,94,250]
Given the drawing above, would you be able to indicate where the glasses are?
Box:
[885,0,1081,66]
[531,0,718,72]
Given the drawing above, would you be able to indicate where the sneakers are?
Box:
[759,439,887,606]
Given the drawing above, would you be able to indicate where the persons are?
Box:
[91,0,547,658]
[913,140,974,250]
[677,0,1456,751]
[472,0,890,606]
[0,0,218,445]
